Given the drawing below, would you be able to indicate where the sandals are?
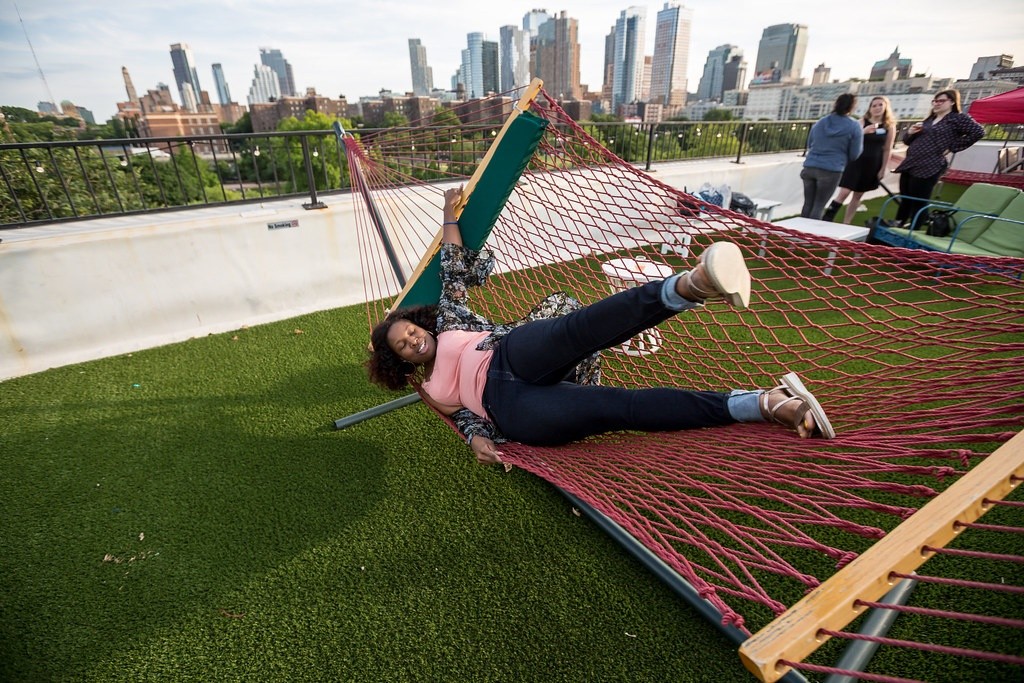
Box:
[686,242,751,309]
[764,372,836,440]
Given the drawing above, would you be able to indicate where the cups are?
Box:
[875,123,886,135]
[916,122,923,127]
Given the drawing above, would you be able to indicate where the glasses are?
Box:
[932,99,951,105]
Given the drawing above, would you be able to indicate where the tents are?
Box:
[949,85,1024,176]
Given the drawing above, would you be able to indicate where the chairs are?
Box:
[999,147,1024,176]
[873,170,1024,280]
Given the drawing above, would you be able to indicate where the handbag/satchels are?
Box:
[925,209,957,237]
[677,182,755,218]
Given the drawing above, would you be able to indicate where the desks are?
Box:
[661,199,782,259]
[602,259,672,355]
[759,216,870,275]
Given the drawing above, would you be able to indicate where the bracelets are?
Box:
[444,221,458,224]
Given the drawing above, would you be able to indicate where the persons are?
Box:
[370,184,835,464]
[822,96,896,224]
[800,94,864,220]
[890,89,985,230]
